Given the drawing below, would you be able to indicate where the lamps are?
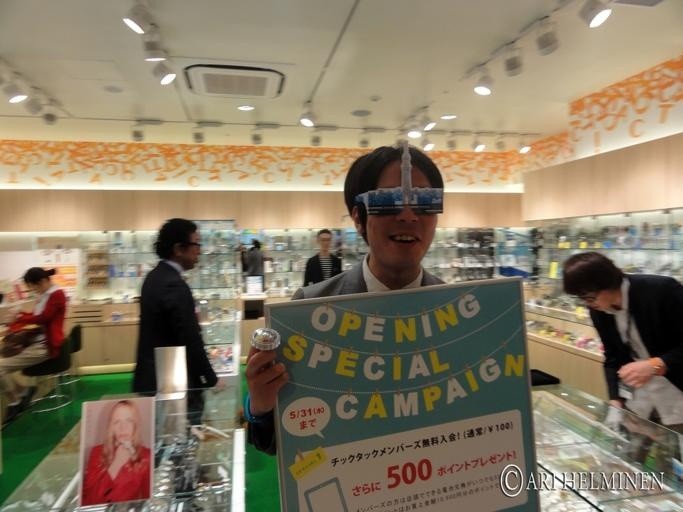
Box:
[3,70,63,125]
[120,4,176,87]
[472,1,611,96]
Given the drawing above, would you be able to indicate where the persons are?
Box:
[562,249,682,477]
[302,228,342,285]
[83,400,151,506]
[132,218,228,510]
[234,240,274,275]
[243,138,454,455]
[0,266,67,424]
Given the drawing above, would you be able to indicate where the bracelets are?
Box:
[245,394,258,424]
[650,356,666,375]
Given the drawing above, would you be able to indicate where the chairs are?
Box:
[20,324,83,413]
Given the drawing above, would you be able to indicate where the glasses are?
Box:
[570,293,597,304]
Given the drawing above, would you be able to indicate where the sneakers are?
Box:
[4,386,37,423]
